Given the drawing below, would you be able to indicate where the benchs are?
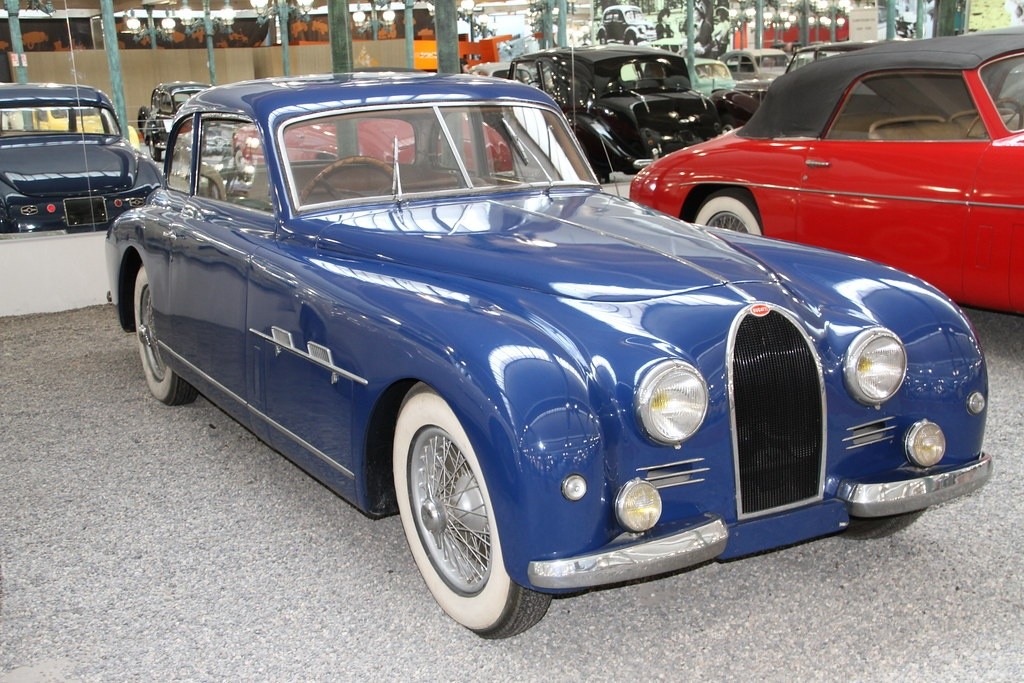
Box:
[269,164,447,209]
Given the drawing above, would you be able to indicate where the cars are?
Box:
[507,44,730,181]
[630,24,1023,319]
[648,37,881,106]
[1,79,165,239]
[103,70,994,639]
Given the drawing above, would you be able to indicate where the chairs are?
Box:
[635,62,667,89]
[869,107,1021,141]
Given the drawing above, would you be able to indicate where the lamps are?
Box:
[126,0,474,30]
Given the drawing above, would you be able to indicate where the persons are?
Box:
[655,13,678,53]
[711,8,731,60]
[692,7,712,58]
